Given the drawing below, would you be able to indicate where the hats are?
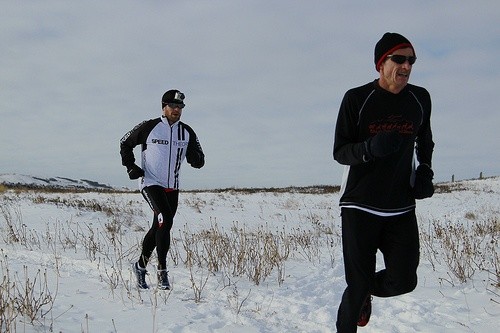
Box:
[162,90,183,108]
[374,31,416,74]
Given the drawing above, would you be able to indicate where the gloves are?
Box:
[189,149,201,163]
[410,165,435,199]
[126,163,144,180]
[371,133,403,158]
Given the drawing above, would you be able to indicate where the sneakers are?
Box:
[157,271,171,290]
[357,294,373,327]
[132,262,149,289]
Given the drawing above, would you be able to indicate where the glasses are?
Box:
[168,103,185,108]
[386,54,418,65]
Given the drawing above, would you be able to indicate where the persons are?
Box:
[333,32,435,333]
[120,89,205,289]
